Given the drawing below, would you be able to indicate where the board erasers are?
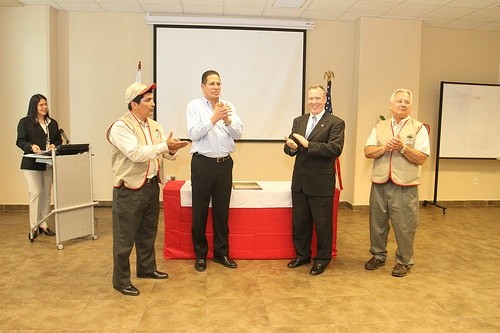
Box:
[496,157,500,160]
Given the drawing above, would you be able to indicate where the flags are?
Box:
[324,81,344,191]
[136,69,143,84]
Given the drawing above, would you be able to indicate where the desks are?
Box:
[163,180,341,260]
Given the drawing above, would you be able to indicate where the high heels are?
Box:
[29,231,37,240]
[38,227,55,236]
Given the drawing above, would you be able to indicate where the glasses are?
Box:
[206,81,223,87]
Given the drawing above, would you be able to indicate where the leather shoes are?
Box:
[195,258,206,271]
[287,257,312,268]
[137,271,168,279]
[309,262,328,276]
[213,256,237,268]
[113,284,139,296]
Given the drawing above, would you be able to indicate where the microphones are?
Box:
[59,129,70,143]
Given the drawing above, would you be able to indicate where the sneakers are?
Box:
[364,256,386,270]
[392,263,411,277]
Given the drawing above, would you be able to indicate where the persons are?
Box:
[16,93,62,241]
[184,70,242,271]
[364,89,430,277]
[106,82,188,295]
[284,86,345,276]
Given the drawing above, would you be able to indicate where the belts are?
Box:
[194,153,230,163]
[144,176,159,184]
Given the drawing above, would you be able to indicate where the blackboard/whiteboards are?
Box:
[436,80,500,160]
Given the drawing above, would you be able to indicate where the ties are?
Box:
[306,117,317,140]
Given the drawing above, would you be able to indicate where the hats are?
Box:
[125,82,156,104]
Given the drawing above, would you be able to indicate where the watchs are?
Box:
[401,145,406,155]
